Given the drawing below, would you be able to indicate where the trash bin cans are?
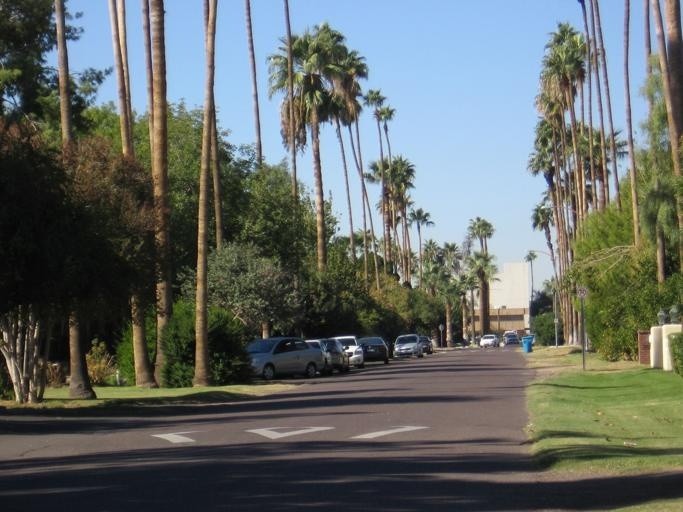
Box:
[522,335,533,352]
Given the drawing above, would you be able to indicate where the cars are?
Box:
[473,330,520,348]
[243,332,435,383]
